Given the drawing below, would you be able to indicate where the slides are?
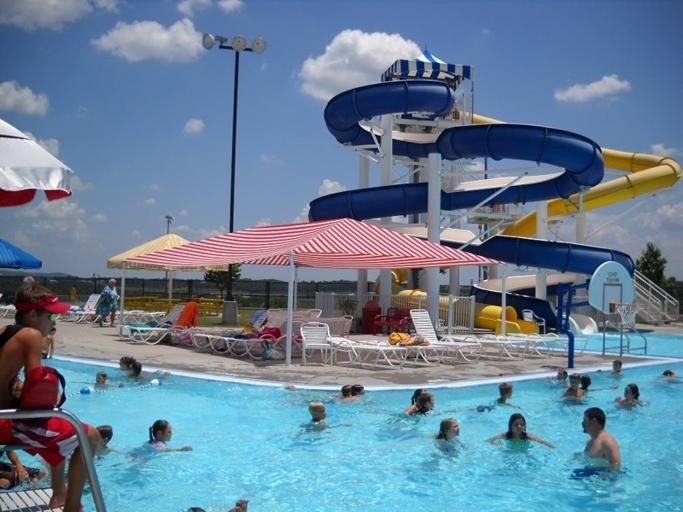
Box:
[309,82,683,336]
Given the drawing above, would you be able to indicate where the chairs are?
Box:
[0,293,103,326]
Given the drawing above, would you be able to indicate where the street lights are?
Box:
[164,215,174,298]
[202,32,267,325]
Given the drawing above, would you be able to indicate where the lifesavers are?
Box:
[568,467,632,481]
[98,299,111,315]
[496,433,544,452]
[559,391,592,404]
[80,369,164,394]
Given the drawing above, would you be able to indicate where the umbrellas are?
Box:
[107,233,229,301]
[0,117,74,207]
[0,238,42,270]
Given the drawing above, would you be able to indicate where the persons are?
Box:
[434,419,461,441]
[119,355,146,379]
[581,408,621,469]
[496,382,520,409]
[398,335,431,347]
[2,277,101,511]
[70,286,77,301]
[95,371,112,391]
[404,388,426,417]
[97,279,120,328]
[2,444,45,490]
[187,499,248,512]
[149,420,189,451]
[95,425,113,455]
[415,392,437,415]
[307,384,366,433]
[552,361,677,409]
[41,328,56,359]
[486,413,557,450]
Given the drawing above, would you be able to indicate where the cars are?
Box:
[185,293,223,305]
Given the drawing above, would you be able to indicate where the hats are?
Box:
[15,292,70,313]
